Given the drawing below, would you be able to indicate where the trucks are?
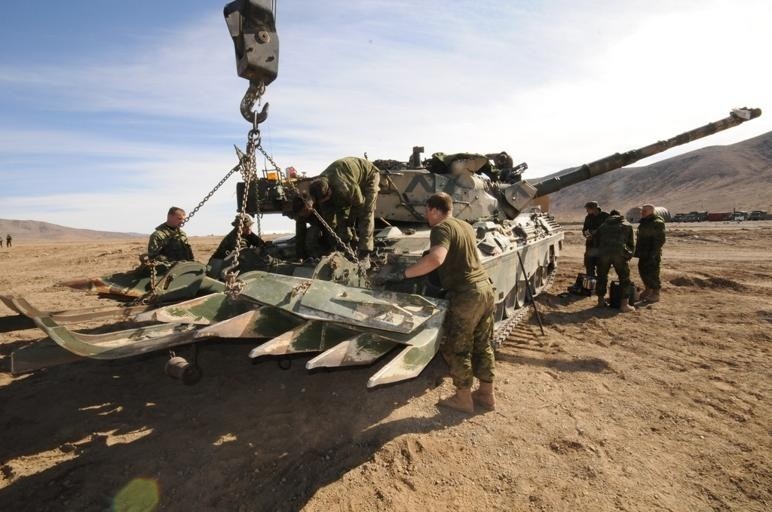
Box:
[672,208,769,222]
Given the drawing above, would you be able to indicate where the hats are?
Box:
[583,200,601,210]
[231,212,255,229]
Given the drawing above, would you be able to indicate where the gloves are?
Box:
[384,270,408,283]
[422,250,431,258]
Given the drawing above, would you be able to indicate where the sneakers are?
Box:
[356,250,372,271]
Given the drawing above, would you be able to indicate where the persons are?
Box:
[591,209,635,312]
[0,237,4,246]
[149,207,194,263]
[632,204,665,304]
[374,190,498,412]
[208,213,266,262]
[7,234,12,247]
[309,156,380,267]
[294,191,338,257]
[582,201,609,277]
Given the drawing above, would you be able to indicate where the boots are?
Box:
[470,381,497,412]
[437,386,475,414]
[597,296,611,307]
[639,286,661,304]
[619,297,636,314]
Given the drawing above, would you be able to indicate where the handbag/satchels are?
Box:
[618,243,634,262]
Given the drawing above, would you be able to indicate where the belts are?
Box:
[445,276,494,296]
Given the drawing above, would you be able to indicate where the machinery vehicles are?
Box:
[0,103,767,402]
